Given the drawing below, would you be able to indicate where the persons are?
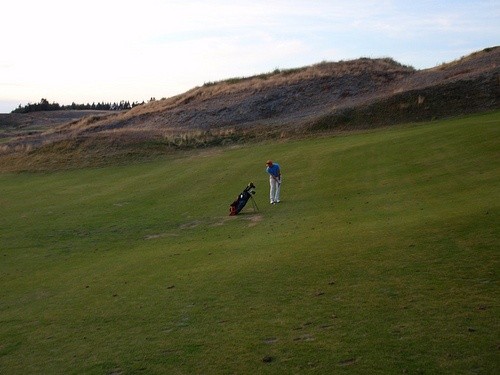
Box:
[265,159,282,204]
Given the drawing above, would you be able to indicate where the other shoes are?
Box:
[270,202,275,204]
[274,200,280,202]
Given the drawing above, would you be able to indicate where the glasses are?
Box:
[266,162,271,165]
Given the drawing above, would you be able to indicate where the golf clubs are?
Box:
[247,183,256,195]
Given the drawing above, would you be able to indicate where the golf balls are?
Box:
[274,203,275,204]
